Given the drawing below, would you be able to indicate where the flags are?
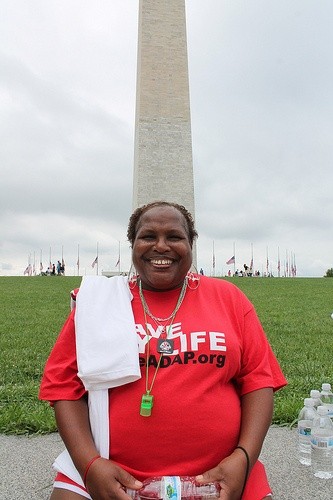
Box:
[22,263,50,277]
[60,256,119,272]
[275,261,297,276]
[215,255,270,268]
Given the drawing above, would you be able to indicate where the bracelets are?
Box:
[232,446,249,498]
[82,456,100,489]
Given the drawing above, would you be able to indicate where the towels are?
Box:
[51,274,142,486]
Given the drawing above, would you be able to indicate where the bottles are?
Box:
[127,476,219,500]
[297,384,333,479]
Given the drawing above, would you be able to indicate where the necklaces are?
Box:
[134,278,201,354]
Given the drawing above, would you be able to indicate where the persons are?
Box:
[198,264,273,277]
[43,262,63,276]
[36,200,287,500]
